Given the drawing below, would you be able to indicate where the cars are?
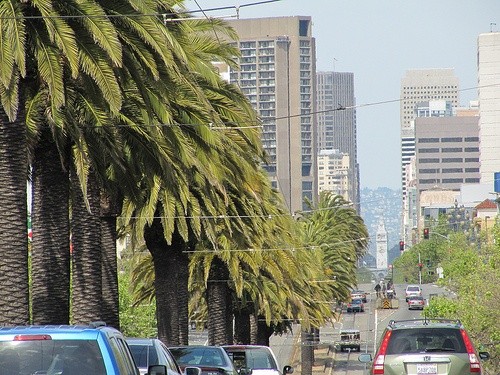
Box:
[168,345,253,375]
[125,336,202,375]
[212,345,295,375]
[346,289,368,313]
[406,295,426,310]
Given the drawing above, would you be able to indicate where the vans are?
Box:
[339,330,361,352]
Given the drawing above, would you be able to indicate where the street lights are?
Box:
[485,215,490,231]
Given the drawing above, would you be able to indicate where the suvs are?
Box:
[0,321,168,375]
[404,285,423,302]
[358,317,491,375]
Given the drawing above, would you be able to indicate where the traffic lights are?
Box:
[400,241,404,250]
[424,228,429,239]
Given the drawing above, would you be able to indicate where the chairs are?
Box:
[441,339,460,352]
[394,338,411,351]
